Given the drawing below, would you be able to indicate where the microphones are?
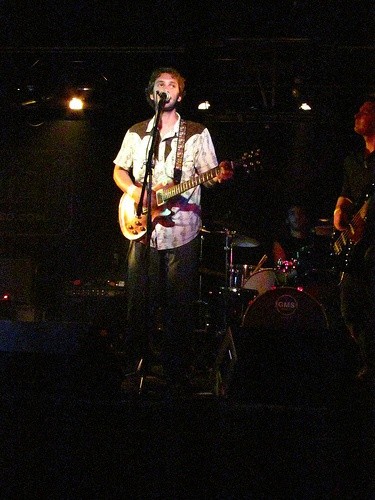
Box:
[157,89,171,102]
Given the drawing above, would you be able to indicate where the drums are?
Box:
[239,267,331,330]
[291,244,327,278]
[220,262,262,295]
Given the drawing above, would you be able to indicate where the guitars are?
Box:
[117,143,273,240]
[324,190,374,273]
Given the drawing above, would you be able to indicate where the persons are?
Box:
[333,93,375,381]
[272,205,312,268]
[113,68,234,382]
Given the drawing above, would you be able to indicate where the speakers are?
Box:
[211,325,351,404]
[0,318,106,400]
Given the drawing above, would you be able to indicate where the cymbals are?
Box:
[200,230,260,248]
[309,224,333,236]
